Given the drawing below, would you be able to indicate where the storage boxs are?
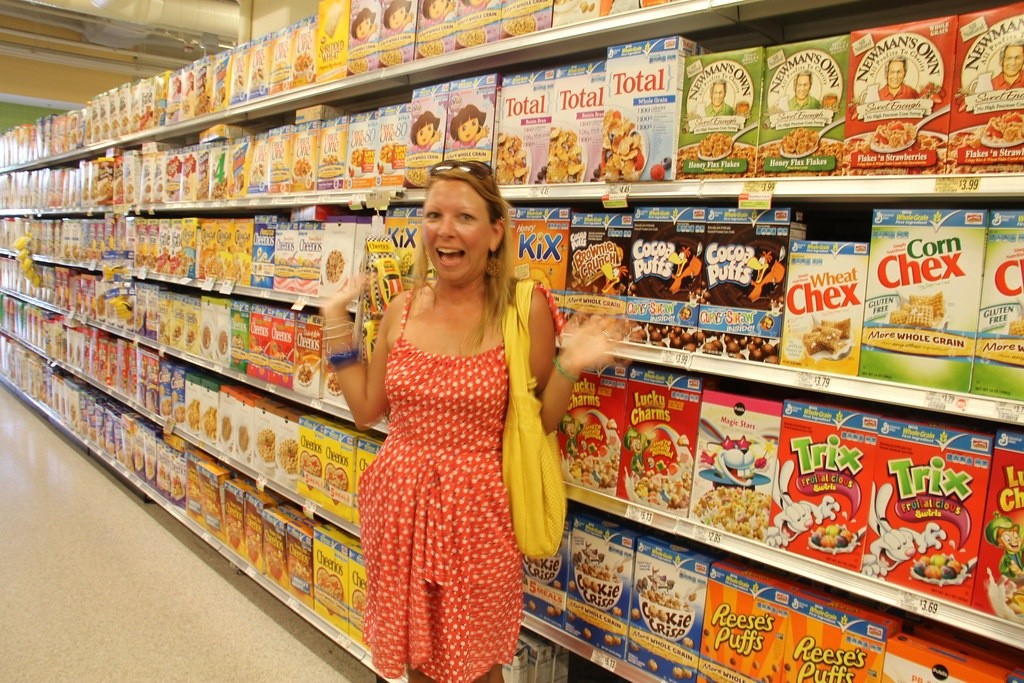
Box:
[0,0,1024,683]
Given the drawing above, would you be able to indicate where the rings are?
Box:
[602,330,609,336]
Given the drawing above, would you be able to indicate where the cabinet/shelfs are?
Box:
[677,0,1024,683]
[42,147,109,454]
[0,164,37,405]
[226,64,403,683]
[19,157,76,420]
[143,107,292,576]
[85,130,194,503]
[383,0,780,683]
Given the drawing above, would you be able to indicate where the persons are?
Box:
[317,160,628,683]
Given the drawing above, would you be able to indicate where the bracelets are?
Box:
[320,321,354,340]
[554,357,579,382]
[328,347,359,363]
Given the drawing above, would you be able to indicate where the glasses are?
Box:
[431,160,493,180]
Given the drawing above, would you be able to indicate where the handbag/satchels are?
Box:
[500,280,568,560]
